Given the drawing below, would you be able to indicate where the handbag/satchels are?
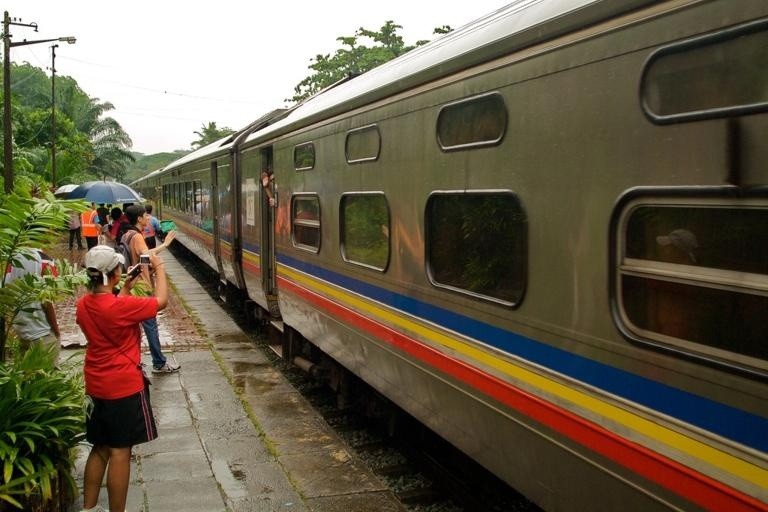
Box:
[116,219,132,242]
[138,362,154,385]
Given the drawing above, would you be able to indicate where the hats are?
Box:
[108,208,122,217]
[101,223,113,235]
[84,245,126,277]
[655,229,697,255]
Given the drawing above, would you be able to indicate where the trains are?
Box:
[127,0,768,512]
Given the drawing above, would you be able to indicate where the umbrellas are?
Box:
[52,179,148,206]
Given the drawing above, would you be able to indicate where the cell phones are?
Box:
[126,263,142,282]
[140,254,151,266]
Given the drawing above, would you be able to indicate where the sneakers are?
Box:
[79,504,111,512]
[68,246,86,250]
[151,363,182,374]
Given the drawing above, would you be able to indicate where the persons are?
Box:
[258,170,279,208]
[1,200,182,376]
[72,245,169,512]
[188,182,233,234]
[378,220,388,244]
[648,224,706,338]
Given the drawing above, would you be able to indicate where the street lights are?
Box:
[1,11,77,195]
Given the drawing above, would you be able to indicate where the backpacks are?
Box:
[117,231,136,275]
